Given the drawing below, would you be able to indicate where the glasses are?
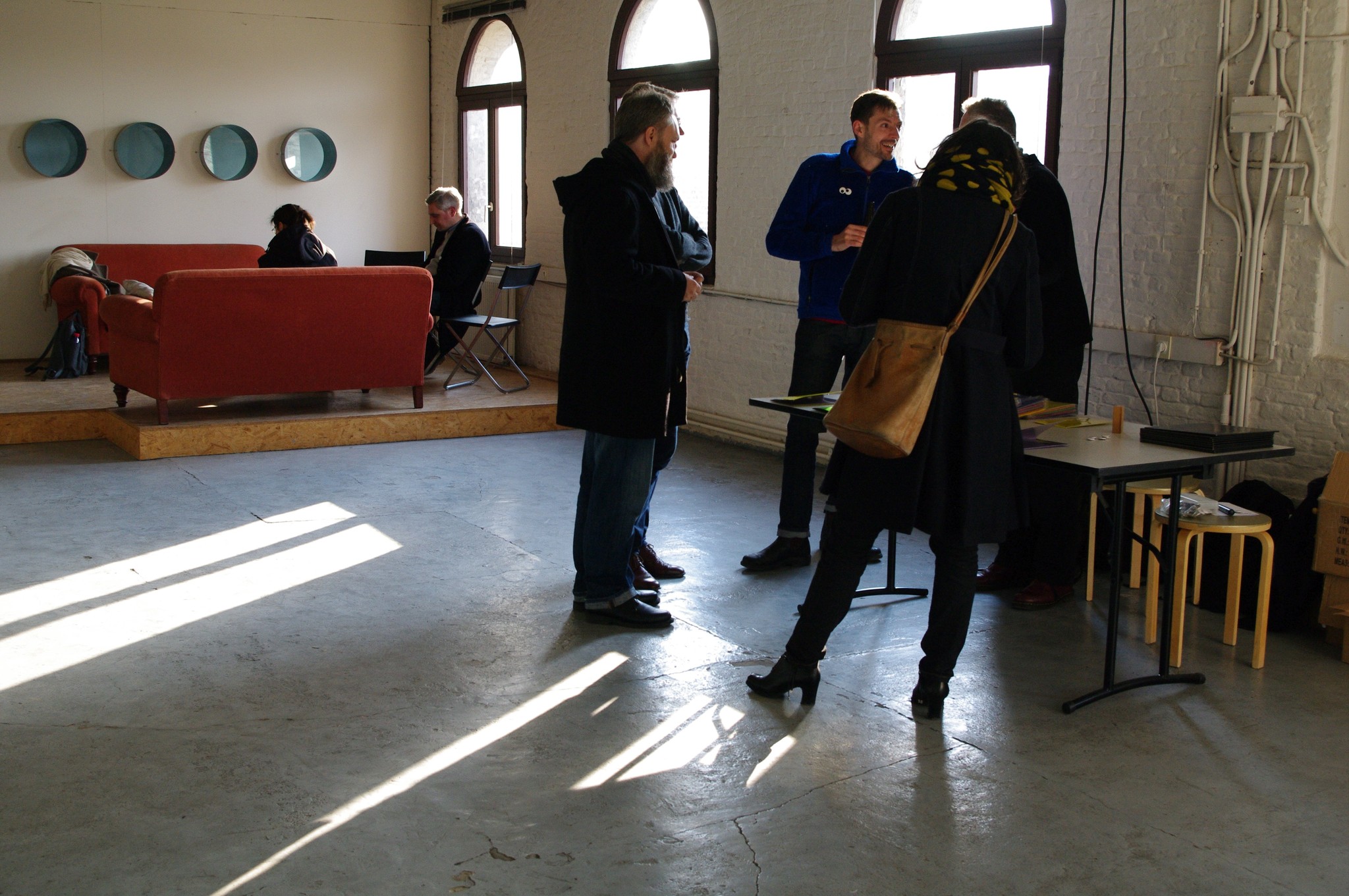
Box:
[272,225,278,235]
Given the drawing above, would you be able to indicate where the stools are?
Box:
[1087,477,1204,602]
[1154,506,1274,669]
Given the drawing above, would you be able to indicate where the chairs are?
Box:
[425,260,493,376]
[364,249,426,266]
[441,263,542,393]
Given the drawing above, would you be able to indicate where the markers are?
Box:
[1218,504,1234,516]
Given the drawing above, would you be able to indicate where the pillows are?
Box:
[123,280,154,300]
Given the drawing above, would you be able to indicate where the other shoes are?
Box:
[868,547,885,562]
[741,537,812,570]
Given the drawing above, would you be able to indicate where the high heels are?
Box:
[745,651,821,707]
[912,673,949,719]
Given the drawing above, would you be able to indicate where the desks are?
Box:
[749,394,1297,716]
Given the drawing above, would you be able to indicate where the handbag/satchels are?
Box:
[823,320,950,458]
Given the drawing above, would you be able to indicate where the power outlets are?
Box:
[1153,335,1171,360]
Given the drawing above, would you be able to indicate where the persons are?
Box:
[622,82,713,592]
[959,97,1095,611]
[741,89,916,572]
[419,186,492,375]
[552,90,705,628]
[258,204,338,269]
[745,117,1041,721]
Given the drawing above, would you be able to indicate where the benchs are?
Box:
[97,266,430,412]
[49,244,266,373]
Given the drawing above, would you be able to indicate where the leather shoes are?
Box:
[631,554,660,588]
[976,562,1005,590]
[639,544,685,579]
[633,588,660,607]
[571,588,673,630]
[1010,578,1071,609]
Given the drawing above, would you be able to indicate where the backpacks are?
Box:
[43,317,87,377]
